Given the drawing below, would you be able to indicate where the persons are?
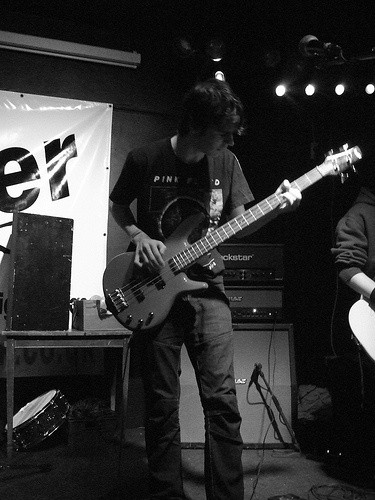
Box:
[108,78,302,500]
[329,142,375,309]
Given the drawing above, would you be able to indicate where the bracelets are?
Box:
[130,229,142,240]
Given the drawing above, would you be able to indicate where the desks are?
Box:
[1,327,136,460]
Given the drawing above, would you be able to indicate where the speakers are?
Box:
[178,323,298,449]
[7,209,74,332]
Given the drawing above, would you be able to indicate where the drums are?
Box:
[4,389,74,451]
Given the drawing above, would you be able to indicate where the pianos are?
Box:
[213,243,288,323]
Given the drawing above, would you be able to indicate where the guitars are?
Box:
[102,143,363,331]
[348,275,375,361]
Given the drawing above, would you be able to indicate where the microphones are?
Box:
[250,363,261,387]
